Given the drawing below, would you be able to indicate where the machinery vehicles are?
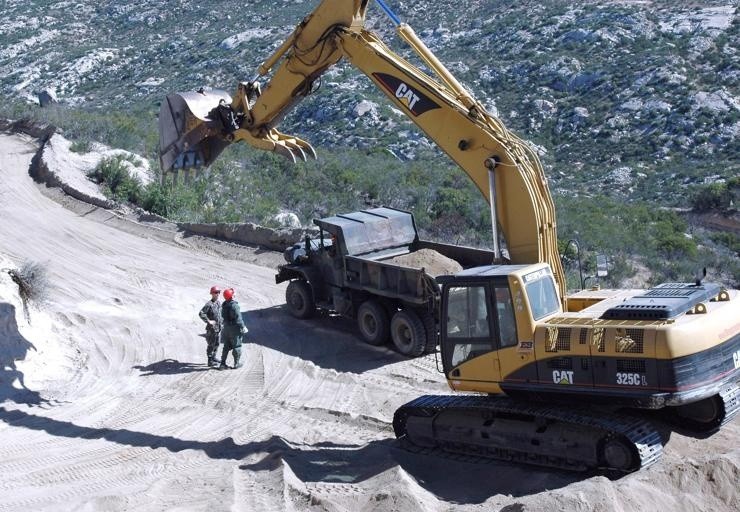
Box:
[158,0,740,478]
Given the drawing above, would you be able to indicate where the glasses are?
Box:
[214,292,220,294]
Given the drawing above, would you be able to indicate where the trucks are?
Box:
[275,203,500,359]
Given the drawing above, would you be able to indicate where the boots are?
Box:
[234,361,242,368]
[220,363,231,369]
[214,358,222,362]
[208,358,217,365]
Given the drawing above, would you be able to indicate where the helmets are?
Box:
[210,286,221,294]
[223,288,235,300]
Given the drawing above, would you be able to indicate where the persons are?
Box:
[221,288,247,370]
[198,285,221,366]
[474,287,513,347]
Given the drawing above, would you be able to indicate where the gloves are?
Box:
[241,326,248,333]
